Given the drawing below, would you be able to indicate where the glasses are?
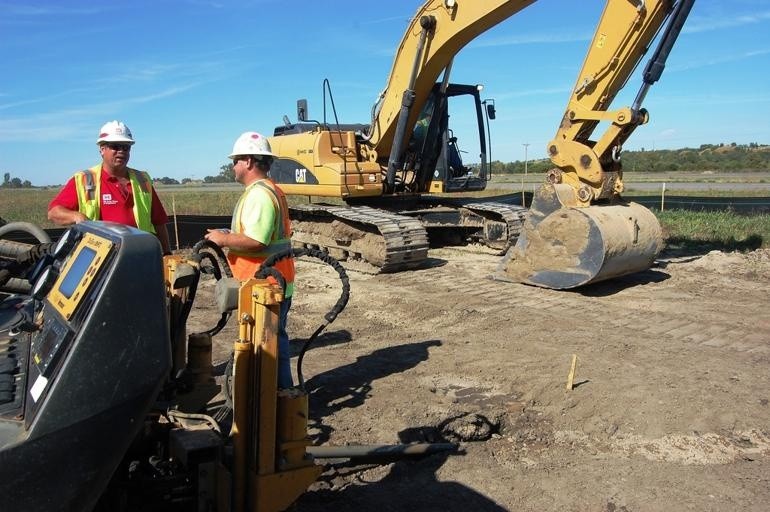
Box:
[105,143,130,151]
[233,157,247,165]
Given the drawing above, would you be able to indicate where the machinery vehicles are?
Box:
[268,0,696,289]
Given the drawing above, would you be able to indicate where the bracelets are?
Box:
[164,252,171,255]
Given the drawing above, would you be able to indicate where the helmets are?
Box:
[96,121,135,144]
[228,132,278,160]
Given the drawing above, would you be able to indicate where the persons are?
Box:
[204,131,294,389]
[48,120,172,257]
[413,108,465,177]
